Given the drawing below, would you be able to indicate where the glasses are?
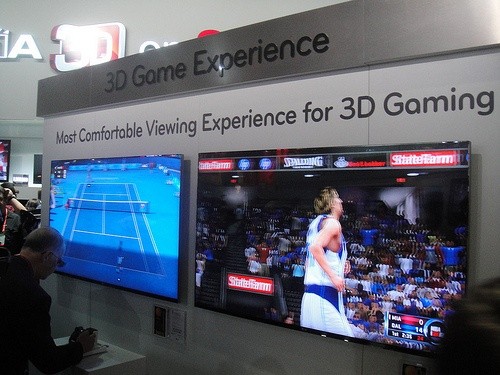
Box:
[49,252,65,266]
[15,191,19,194]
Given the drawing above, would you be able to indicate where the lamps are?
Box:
[12,173,29,188]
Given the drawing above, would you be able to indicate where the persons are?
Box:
[437,278,500,375]
[196,185,465,337]
[0,183,41,255]
[0,226,97,375]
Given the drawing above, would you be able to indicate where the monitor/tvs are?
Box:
[33,154,42,184]
[194,140,472,357]
[49,153,184,302]
[0,140,11,182]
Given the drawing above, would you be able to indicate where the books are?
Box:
[82,345,107,356]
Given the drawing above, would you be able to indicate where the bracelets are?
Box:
[7,197,15,202]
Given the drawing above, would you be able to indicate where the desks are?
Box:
[29,334,147,375]
